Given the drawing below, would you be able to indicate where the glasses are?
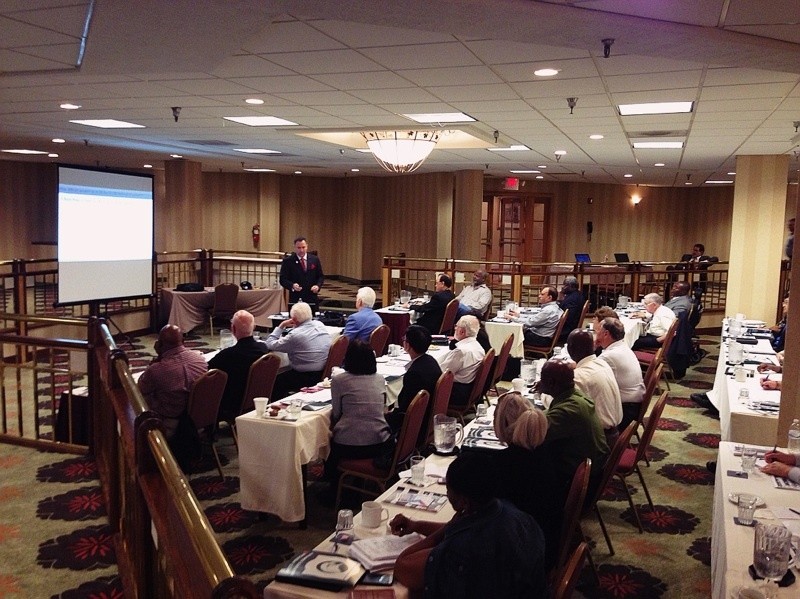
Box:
[644,302,654,307]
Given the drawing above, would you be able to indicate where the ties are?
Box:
[301,258,305,271]
[690,258,696,262]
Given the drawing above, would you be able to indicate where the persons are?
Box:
[666,244,719,301]
[757,349,785,375]
[760,377,782,391]
[769,292,792,353]
[138,269,495,479]
[785,217,796,258]
[389,276,694,599]
[760,450,800,484]
[280,238,324,318]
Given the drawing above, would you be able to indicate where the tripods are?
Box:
[102,303,135,352]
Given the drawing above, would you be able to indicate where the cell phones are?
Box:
[357,572,394,586]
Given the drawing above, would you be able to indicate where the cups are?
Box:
[410,456,426,480]
[617,299,644,315]
[362,501,389,526]
[753,523,799,581]
[512,378,525,393]
[728,321,742,336]
[728,343,750,364]
[332,366,339,376]
[534,358,547,373]
[738,388,749,404]
[736,313,746,321]
[290,399,303,416]
[423,293,429,301]
[735,370,747,382]
[477,404,488,416]
[253,398,268,415]
[433,416,464,452]
[737,494,757,524]
[394,299,400,306]
[191,350,203,357]
[553,346,561,355]
[389,343,404,361]
[337,509,353,530]
[253,331,260,341]
[742,448,757,474]
[281,312,288,319]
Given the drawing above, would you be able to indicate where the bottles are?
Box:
[787,419,800,454]
[605,254,608,261]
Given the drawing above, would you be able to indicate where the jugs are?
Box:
[400,290,411,304]
[619,296,632,307]
[220,329,235,350]
[521,360,539,383]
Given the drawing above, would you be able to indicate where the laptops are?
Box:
[614,253,629,266]
[574,253,592,263]
[287,302,316,319]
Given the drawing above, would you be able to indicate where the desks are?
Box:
[57,283,800,599]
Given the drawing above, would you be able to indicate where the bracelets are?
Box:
[774,381,778,390]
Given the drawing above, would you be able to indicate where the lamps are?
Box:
[361,129,442,173]
